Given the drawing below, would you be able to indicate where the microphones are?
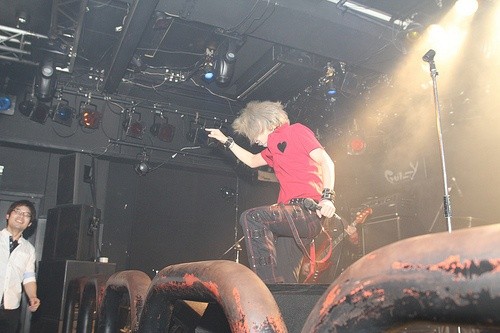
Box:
[304,199,342,221]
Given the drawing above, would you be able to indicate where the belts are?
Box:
[289,198,318,205]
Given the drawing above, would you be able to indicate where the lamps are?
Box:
[134,159,150,176]
[35,63,57,102]
[19,91,51,125]
[16,0,30,30]
[405,23,423,43]
[341,0,405,27]
[0,94,17,116]
[328,78,336,95]
[186,119,209,145]
[122,109,145,140]
[340,72,363,97]
[327,0,341,4]
[202,55,217,84]
[51,99,73,128]
[87,66,105,82]
[218,42,240,85]
[220,185,236,201]
[344,135,367,156]
[149,113,174,144]
[77,101,100,129]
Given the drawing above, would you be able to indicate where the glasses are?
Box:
[13,209,32,218]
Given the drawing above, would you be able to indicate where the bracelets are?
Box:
[321,194,336,202]
[224,137,235,148]
[322,187,335,197]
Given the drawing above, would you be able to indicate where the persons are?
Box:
[206,100,336,283]
[0,199,41,333]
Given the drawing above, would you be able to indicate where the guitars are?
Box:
[298,208,372,284]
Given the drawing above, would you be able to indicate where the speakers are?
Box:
[361,215,424,257]
[29,152,117,333]
